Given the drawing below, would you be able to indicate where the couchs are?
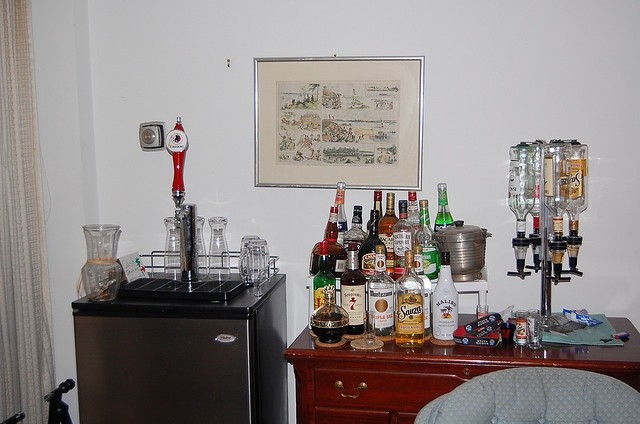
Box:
[414,367,629,423]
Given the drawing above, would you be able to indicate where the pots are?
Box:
[432,220,492,282]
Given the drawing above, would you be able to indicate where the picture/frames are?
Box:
[251,55,425,192]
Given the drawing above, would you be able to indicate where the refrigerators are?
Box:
[71,272,289,424]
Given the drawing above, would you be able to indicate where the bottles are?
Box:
[515,310,529,347]
[394,249,426,351]
[338,242,368,336]
[330,181,349,249]
[432,251,460,341]
[406,190,421,241]
[410,243,433,342]
[526,141,543,235]
[508,144,537,237]
[433,182,454,231]
[308,204,346,277]
[367,190,384,237]
[309,284,350,344]
[413,198,439,282]
[366,243,397,337]
[358,209,387,280]
[311,240,338,317]
[560,143,589,237]
[542,143,572,240]
[378,192,400,278]
[391,199,414,281]
[340,202,371,270]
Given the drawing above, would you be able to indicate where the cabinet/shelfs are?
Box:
[285,313,639,424]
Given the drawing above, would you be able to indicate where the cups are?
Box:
[193,215,208,281]
[237,238,271,285]
[240,235,261,283]
[162,216,186,283]
[363,308,376,346]
[206,214,232,281]
[526,314,544,351]
